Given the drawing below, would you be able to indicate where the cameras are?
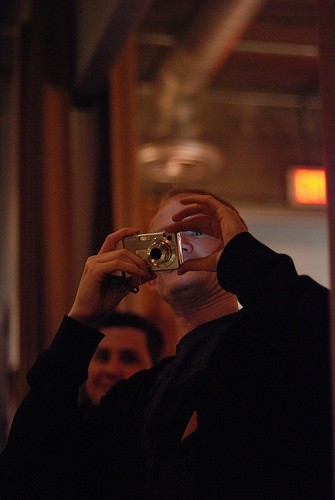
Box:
[123,231,183,271]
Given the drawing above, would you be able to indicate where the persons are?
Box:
[0,182,335,500]
[81,310,166,407]
